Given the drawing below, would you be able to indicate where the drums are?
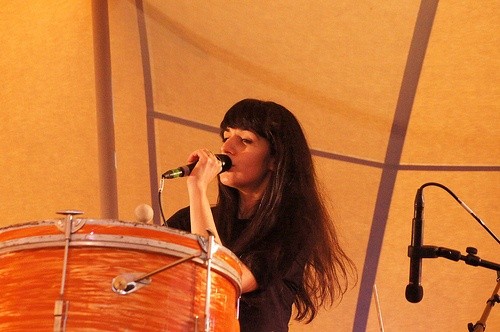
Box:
[0,210,243,332]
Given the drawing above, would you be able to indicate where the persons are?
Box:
[163,98,358,331]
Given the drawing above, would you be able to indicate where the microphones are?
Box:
[162,154,233,179]
[406,188,423,303]
